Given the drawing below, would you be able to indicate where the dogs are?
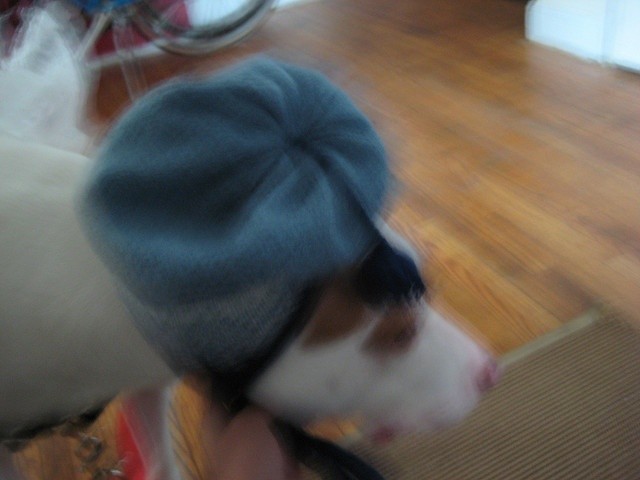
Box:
[0,58,498,480]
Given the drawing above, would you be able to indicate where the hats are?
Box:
[78,54,426,418]
